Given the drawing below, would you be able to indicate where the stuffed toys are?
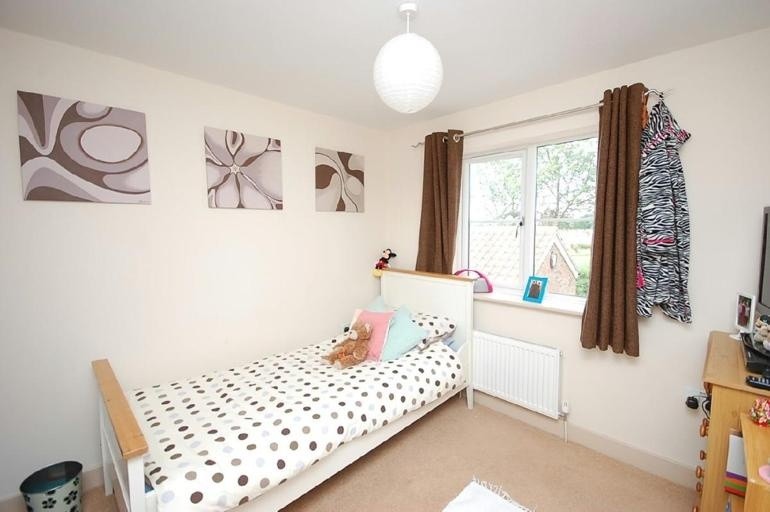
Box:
[374,248,397,277]
[319,319,375,371]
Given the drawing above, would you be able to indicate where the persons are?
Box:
[737,299,750,326]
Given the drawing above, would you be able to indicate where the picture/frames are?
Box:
[521,276,548,304]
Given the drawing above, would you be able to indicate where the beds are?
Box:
[90,265,475,512]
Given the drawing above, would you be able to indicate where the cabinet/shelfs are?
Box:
[695,325,770,512]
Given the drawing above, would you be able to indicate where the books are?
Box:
[723,433,749,500]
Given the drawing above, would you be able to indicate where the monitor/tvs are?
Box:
[755,206,770,315]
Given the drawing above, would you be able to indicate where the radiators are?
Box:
[475,329,570,421]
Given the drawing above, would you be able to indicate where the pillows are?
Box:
[348,296,457,362]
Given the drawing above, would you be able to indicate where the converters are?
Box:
[686,397,698,410]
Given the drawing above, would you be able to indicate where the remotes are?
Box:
[746,375,770,389]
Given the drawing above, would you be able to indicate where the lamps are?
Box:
[373,3,444,117]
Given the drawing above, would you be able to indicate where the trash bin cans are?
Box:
[20,461,82,512]
